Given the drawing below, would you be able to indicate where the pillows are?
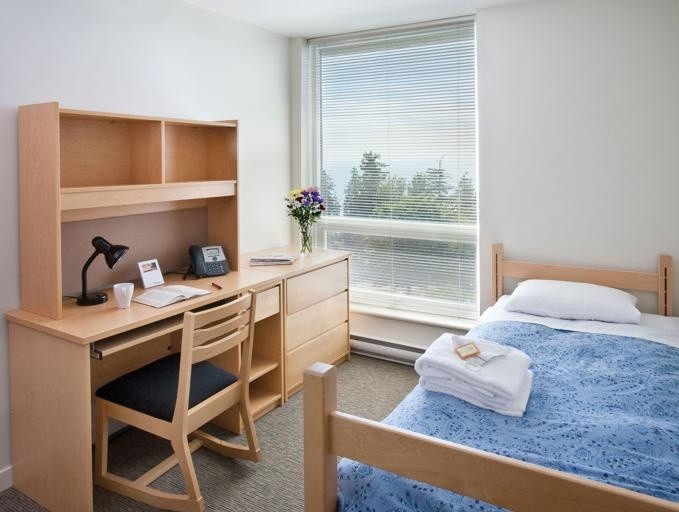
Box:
[506,278,642,324]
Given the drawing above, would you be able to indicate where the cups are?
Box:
[113,282,135,309]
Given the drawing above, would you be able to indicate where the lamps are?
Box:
[76,237,128,307]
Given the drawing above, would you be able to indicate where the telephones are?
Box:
[189,245,229,277]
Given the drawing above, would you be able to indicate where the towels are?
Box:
[415,333,534,419]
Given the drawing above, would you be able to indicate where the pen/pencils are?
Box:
[212,283,222,289]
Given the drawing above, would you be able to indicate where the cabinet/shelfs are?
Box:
[17,102,240,320]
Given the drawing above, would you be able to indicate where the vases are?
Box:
[298,220,315,255]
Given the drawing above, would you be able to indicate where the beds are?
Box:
[300,242,679,508]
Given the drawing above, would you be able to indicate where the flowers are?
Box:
[285,186,327,247]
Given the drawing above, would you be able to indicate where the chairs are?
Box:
[90,288,262,512]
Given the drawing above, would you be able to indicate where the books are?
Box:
[249,252,297,265]
[130,283,213,308]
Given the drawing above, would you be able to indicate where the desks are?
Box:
[4,250,349,511]
